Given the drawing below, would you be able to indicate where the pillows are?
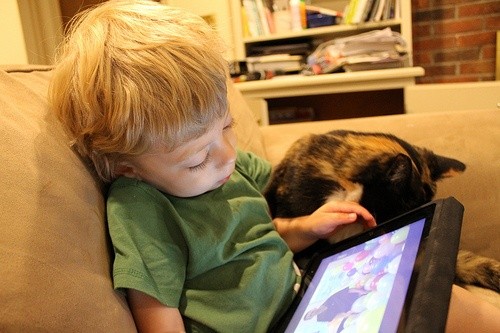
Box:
[416,112,500,260]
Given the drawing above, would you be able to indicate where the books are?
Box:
[239,0,400,36]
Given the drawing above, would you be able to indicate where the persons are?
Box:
[50,0,377,333]
[303,286,367,323]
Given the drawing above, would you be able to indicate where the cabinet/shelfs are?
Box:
[231,0,500,125]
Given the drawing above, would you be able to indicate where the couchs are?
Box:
[0,63,500,333]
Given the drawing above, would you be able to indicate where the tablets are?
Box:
[267,197,444,333]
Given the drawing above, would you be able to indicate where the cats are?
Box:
[262,129,500,294]
